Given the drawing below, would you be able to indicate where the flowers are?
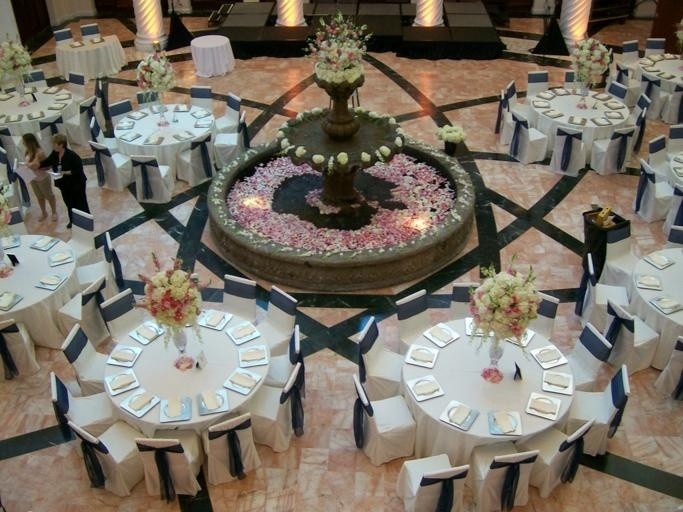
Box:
[438,125,467,143]
[136,51,176,101]
[0,196,11,225]
[130,252,212,349]
[0,32,33,87]
[570,33,610,89]
[675,18,683,46]
[467,252,543,361]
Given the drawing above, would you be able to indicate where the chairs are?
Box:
[607,80,628,103]
[668,125,683,147]
[564,71,582,90]
[36,112,71,157]
[473,441,540,512]
[661,81,683,124]
[352,373,416,466]
[88,141,135,192]
[3,158,31,206]
[396,289,434,355]
[8,207,28,235]
[662,182,683,244]
[515,417,595,498]
[451,283,479,321]
[23,74,33,93]
[256,285,297,357]
[495,89,538,145]
[202,412,261,486]
[61,323,110,397]
[528,291,560,341]
[647,134,670,167]
[215,92,241,133]
[0,319,40,380]
[108,99,132,128]
[89,116,118,154]
[645,39,665,57]
[616,62,642,107]
[599,47,616,91]
[397,454,470,512]
[565,323,613,391]
[76,231,125,291]
[0,127,27,161]
[65,95,97,145]
[507,80,536,112]
[214,110,249,168]
[526,70,548,104]
[176,131,216,187]
[68,72,85,103]
[100,288,143,343]
[190,86,212,112]
[30,71,48,92]
[130,155,172,203]
[134,429,205,504]
[629,93,652,153]
[590,127,636,176]
[50,371,119,438]
[67,420,145,497]
[53,28,74,46]
[602,298,660,376]
[223,274,257,326]
[59,274,106,340]
[567,364,631,456]
[188,274,199,286]
[137,91,159,109]
[623,40,639,62]
[550,126,585,176]
[263,325,305,398]
[66,208,95,265]
[575,253,629,333]
[642,72,669,120]
[238,362,301,452]
[80,23,100,40]
[357,316,404,401]
[94,79,113,132]
[653,336,683,400]
[633,158,674,223]
[0,146,10,190]
[510,111,547,165]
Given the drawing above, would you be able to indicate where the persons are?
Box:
[19,134,59,223]
[29,134,92,228]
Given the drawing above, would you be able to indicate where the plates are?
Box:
[127,110,148,120]
[642,250,676,270]
[143,136,164,145]
[604,100,625,110]
[532,100,550,108]
[639,58,656,66]
[542,371,574,395]
[404,343,439,369]
[525,391,562,421]
[196,389,229,416]
[506,328,537,347]
[223,321,261,345]
[116,121,135,130]
[90,37,105,44]
[568,116,586,126]
[191,109,212,119]
[536,91,556,100]
[107,344,142,368]
[661,54,678,59]
[174,104,193,113]
[239,344,269,369]
[4,114,23,123]
[572,87,588,96]
[642,66,661,72]
[656,72,676,80]
[198,310,233,331]
[422,321,461,347]
[195,119,214,130]
[172,130,196,141]
[541,109,564,119]
[223,367,262,395]
[0,290,24,312]
[673,166,683,176]
[0,94,14,101]
[27,111,45,120]
[646,54,665,62]
[34,270,68,291]
[439,399,481,432]
[590,117,613,127]
[1,233,22,250]
[70,41,85,48]
[105,369,140,395]
[43,86,64,94]
[47,249,74,267]
[673,155,683,164]
[55,93,72,100]
[405,373,446,402]
[29,236,59,252]
[119,130,142,142]
[604,111,625,119]
[530,343,569,370]
[653,274,664,291]
[648,296,683,315]
[592,92,613,101]
[551,88,571,96]
[24,87,38,94]
[150,104,168,114]
[48,103,68,110]
[487,410,523,437]
[128,321,165,346]
[466,317,494,337]
[120,389,161,419]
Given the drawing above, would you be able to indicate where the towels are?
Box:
[39,275,60,285]
[659,71,672,78]
[0,235,14,248]
[49,251,72,262]
[111,350,137,362]
[410,349,434,363]
[529,398,558,415]
[430,325,453,343]
[608,100,624,109]
[229,372,257,389]
[412,380,439,396]
[676,167,683,174]
[640,59,654,66]
[24,87,33,94]
[197,119,211,126]
[573,116,582,124]
[536,349,562,363]
[92,36,102,43]
[167,395,181,418]
[129,391,155,410]
[178,131,193,139]
[200,388,221,410]
[50,103,65,109]
[35,237,51,249]
[0,292,17,308]
[230,324,255,340]
[645,66,658,72]
[122,131,137,140]
[637,275,660,288]
[45,85,58,94]
[241,349,266,362]
[664,52,675,59]
[471,320,487,334]
[178,103,188,111]
[649,54,662,61]
[110,372,135,390]
[0,93,11,100]
[594,93,609,100]
[533,101,549,107]
[448,404,472,425]
[594,117,609,125]
[117,122,133,129]
[9,114,19,122]
[606,112,621,119]
[31,111,42,119]
[152,104,159,113]
[576,87,581,95]
[493,411,516,434]
[206,310,226,327]
[658,297,678,312]
[73,41,83,47]
[674,154,682,161]
[555,87,568,96]
[148,136,159,144]
[129,111,145,118]
[648,252,668,268]
[54,94,69,100]
[508,334,522,342]
[193,110,208,118]
[544,372,570,388]
[137,324,159,342]
[545,110,561,118]
[538,91,553,99]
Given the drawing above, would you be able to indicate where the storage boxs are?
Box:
[583,208,631,287]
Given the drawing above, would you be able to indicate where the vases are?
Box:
[15,84,30,107]
[481,344,503,384]
[157,106,169,126]
[0,240,13,277]
[445,144,457,156]
[173,330,194,370]
[577,89,589,109]
